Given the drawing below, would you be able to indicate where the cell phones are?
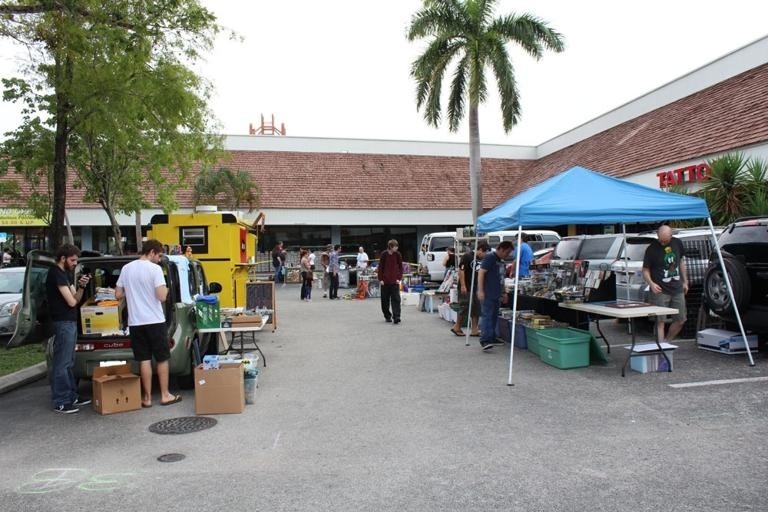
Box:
[77,273,92,284]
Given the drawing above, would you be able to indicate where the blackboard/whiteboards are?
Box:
[246,281,275,325]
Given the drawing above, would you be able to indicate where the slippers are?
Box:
[141,402,152,407]
[451,329,466,336]
[160,393,182,405]
[469,332,481,336]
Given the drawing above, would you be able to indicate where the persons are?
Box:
[114,239,182,408]
[45,243,93,415]
[449,238,492,338]
[271,240,380,301]
[639,224,689,348]
[474,240,514,352]
[508,231,537,281]
[2,246,31,268]
[442,247,459,281]
[376,237,406,324]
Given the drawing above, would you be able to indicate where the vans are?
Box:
[419,230,470,284]
[63,254,228,390]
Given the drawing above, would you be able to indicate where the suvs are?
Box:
[472,222,564,289]
[612,230,727,342]
[574,227,627,312]
[693,215,761,342]
[542,226,593,298]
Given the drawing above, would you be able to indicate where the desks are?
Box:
[556,296,680,377]
[197,310,271,367]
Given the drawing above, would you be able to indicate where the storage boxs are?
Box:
[91,361,142,416]
[79,291,127,334]
[449,303,467,326]
[495,312,677,374]
[192,352,262,415]
[695,328,760,357]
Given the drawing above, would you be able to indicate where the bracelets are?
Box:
[79,283,88,290]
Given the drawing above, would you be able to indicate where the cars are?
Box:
[0,258,48,347]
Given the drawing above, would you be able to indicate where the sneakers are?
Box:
[482,344,493,350]
[72,397,91,406]
[493,338,505,345]
[53,404,80,414]
